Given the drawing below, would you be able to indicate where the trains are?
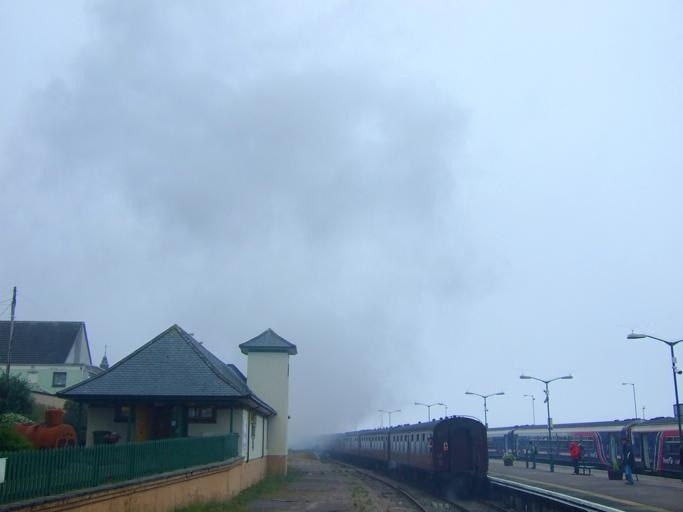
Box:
[487,416,682,480]
[312,414,488,499]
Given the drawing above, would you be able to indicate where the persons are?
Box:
[570,442,580,474]
[530,440,537,468]
[621,438,634,485]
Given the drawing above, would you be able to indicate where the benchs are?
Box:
[575,463,597,476]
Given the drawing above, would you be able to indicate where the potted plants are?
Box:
[500,450,517,467]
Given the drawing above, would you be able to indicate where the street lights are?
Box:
[414,402,444,422]
[520,375,573,472]
[627,333,682,482]
[465,392,505,433]
[377,409,402,428]
[524,394,535,425]
[621,383,638,420]
